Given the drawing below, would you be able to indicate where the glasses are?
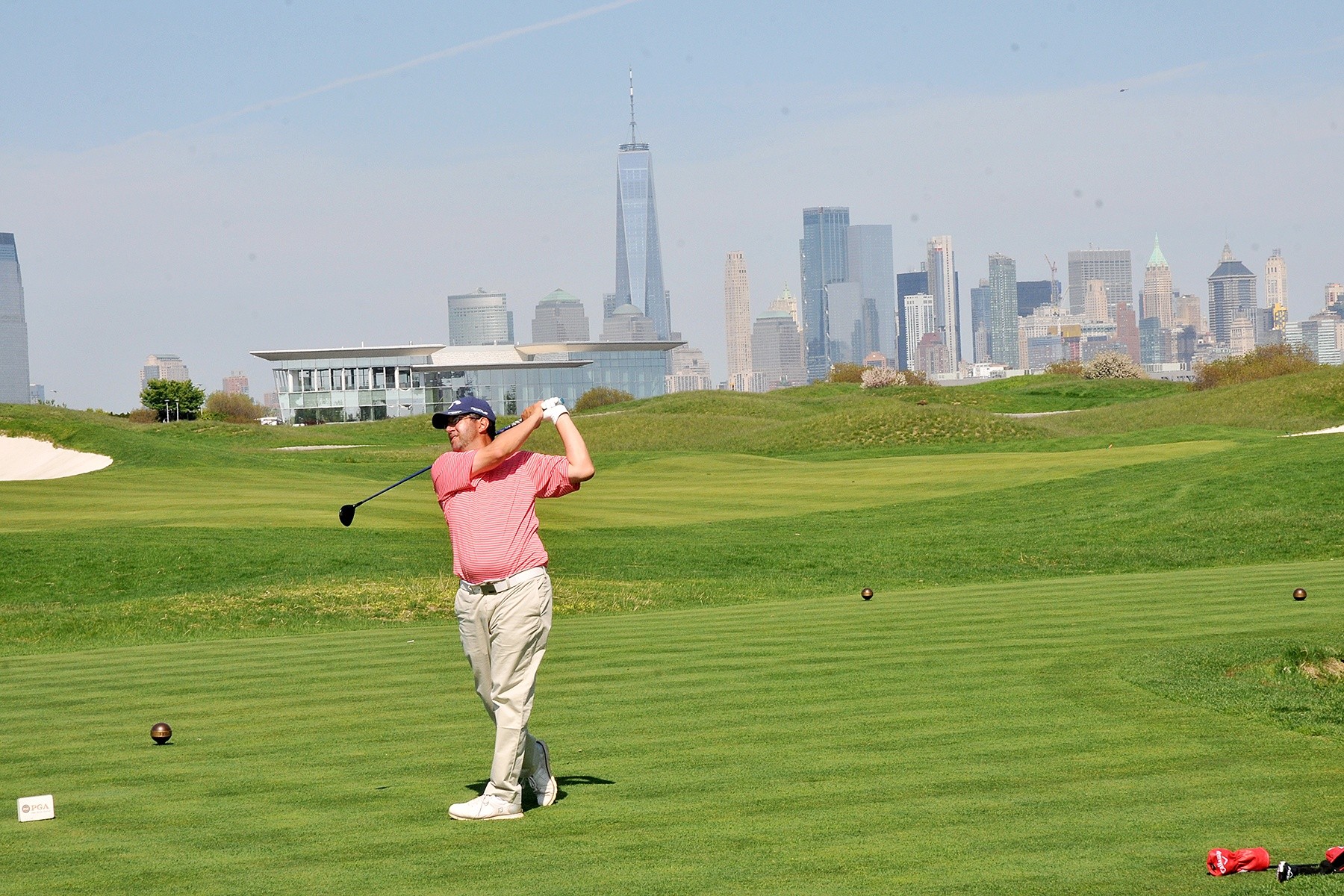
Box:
[444,415,480,429]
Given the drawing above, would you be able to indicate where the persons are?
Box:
[431,397,596,821]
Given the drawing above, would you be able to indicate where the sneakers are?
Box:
[528,741,557,807]
[447,795,524,820]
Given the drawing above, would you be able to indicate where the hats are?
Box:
[432,397,496,429]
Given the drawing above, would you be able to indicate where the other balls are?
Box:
[862,589,873,600]
[1293,588,1306,600]
[150,723,172,744]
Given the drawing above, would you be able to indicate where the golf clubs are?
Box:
[338,397,565,527]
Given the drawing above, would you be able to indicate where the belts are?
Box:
[460,566,545,595]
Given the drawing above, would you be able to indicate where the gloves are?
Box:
[540,397,570,424]
